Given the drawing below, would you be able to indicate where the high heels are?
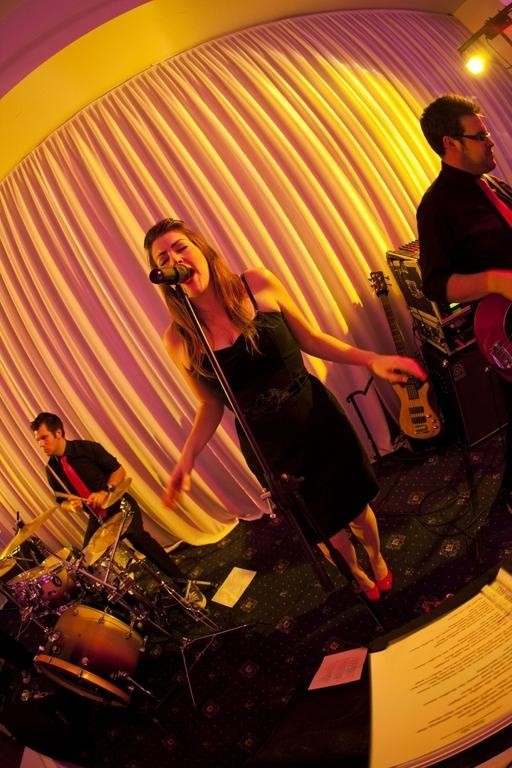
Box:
[363,569,393,601]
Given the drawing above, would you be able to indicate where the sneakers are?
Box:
[183,580,207,609]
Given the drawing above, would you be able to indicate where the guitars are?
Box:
[473,292,512,383]
[368,271,445,441]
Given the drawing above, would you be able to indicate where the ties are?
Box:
[476,177,512,230]
[59,456,108,519]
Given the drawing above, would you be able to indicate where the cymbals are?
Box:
[0,556,17,574]
[87,511,135,553]
[101,477,132,509]
[0,505,59,559]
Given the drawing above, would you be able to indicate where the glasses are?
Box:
[459,133,491,139]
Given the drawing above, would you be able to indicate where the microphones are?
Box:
[149,263,195,284]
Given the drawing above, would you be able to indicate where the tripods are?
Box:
[30,535,256,708]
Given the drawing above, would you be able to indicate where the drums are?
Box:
[6,562,81,623]
[77,539,149,604]
[40,547,100,597]
[32,602,145,707]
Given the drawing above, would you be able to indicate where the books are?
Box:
[370,570,511,768]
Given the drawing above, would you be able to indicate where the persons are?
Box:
[29,412,208,612]
[144,220,427,601]
[418,93,511,310]
